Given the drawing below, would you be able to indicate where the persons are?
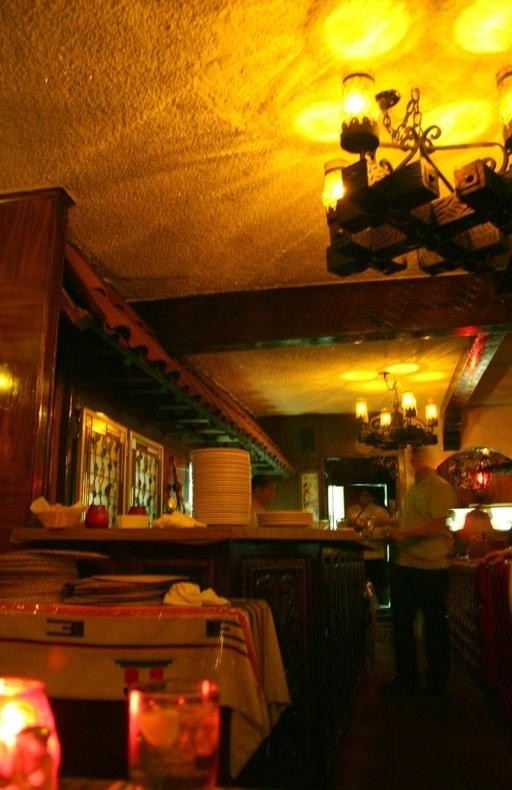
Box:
[374,446,457,695]
[252,474,276,513]
[482,545,512,618]
[345,485,388,605]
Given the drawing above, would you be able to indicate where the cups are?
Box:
[125,680,223,790]
[0,675,66,790]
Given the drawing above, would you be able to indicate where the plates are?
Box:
[188,446,254,525]
[256,510,315,527]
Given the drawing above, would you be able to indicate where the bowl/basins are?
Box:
[35,508,83,530]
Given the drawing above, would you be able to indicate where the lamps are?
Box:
[434,446,512,552]
[351,373,439,447]
[305,53,512,281]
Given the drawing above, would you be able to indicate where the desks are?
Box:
[1,589,288,787]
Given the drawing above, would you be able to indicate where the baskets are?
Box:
[30,507,84,530]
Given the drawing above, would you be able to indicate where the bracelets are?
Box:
[504,546,509,558]
